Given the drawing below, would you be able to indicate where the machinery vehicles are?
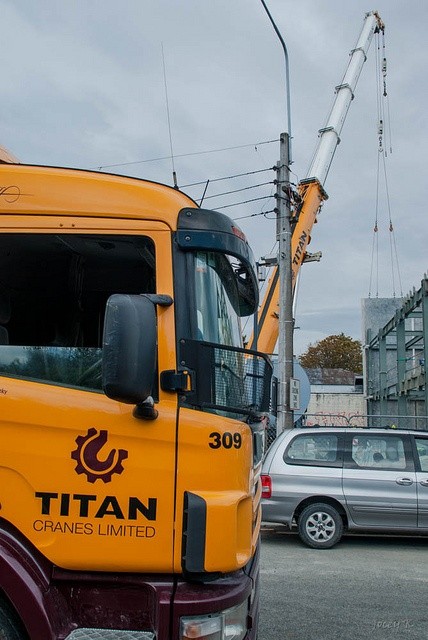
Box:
[242,10,400,405]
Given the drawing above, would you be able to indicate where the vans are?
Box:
[260,427,428,548]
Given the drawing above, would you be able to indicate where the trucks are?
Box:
[1,145,276,640]
